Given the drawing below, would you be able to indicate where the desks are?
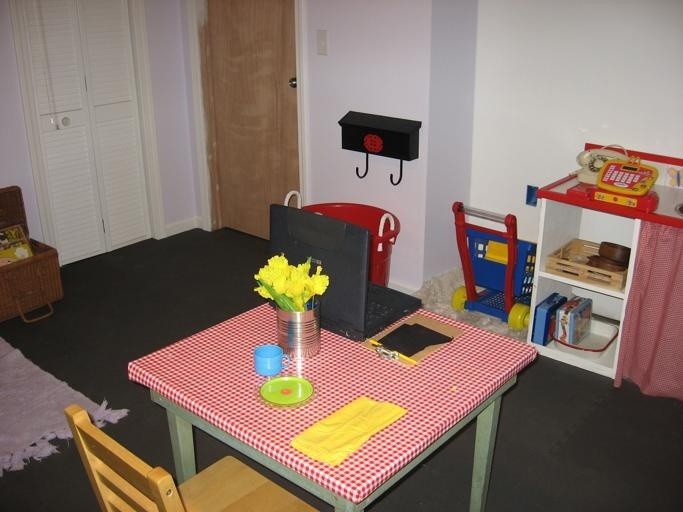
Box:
[124,303,538,512]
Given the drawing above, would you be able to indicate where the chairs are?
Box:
[61,400,318,512]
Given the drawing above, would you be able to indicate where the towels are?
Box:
[289,394,410,469]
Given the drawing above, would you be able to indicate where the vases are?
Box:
[275,298,320,359]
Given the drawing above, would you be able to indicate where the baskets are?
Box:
[2,186,63,323]
[545,238,628,290]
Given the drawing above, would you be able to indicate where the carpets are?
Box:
[409,265,528,346]
[0,331,132,486]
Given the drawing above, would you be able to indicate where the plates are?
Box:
[259,377,314,405]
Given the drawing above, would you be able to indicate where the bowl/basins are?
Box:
[598,241,630,266]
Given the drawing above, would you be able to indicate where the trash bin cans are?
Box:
[298,203,400,287]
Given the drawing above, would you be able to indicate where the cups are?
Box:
[254,345,290,378]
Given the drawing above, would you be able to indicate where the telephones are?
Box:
[576,148,628,185]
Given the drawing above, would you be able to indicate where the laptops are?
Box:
[270,204,421,343]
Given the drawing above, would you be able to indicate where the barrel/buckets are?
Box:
[284,190,401,288]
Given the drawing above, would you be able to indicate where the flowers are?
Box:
[252,251,331,311]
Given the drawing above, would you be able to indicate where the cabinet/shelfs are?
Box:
[8,0,164,267]
[524,183,641,381]
